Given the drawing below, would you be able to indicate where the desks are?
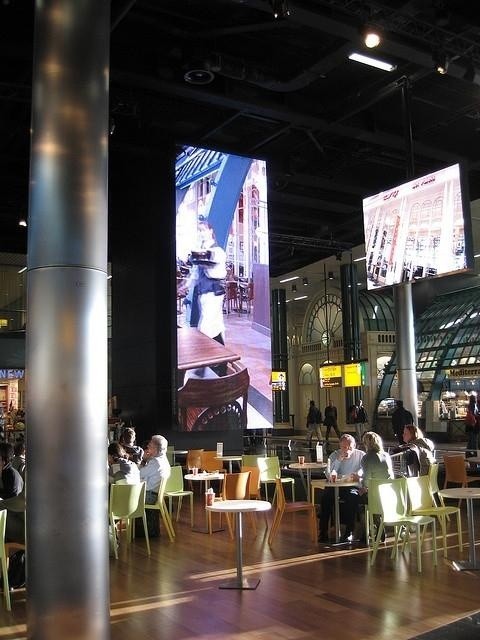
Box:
[177,327,240,372]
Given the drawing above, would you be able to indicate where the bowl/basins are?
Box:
[190,250,211,259]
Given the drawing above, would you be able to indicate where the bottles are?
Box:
[331,469,337,483]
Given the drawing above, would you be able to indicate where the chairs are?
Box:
[178,368,250,431]
[176,254,253,320]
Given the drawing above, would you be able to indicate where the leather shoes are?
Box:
[319,534,360,542]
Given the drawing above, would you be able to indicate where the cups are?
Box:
[205,492,216,506]
[298,456,305,464]
[192,468,199,477]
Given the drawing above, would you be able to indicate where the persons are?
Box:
[349,399,369,442]
[463,394,479,458]
[323,399,342,441]
[0,424,172,594]
[189,219,228,378]
[391,400,414,446]
[303,399,325,449]
[318,424,436,542]
